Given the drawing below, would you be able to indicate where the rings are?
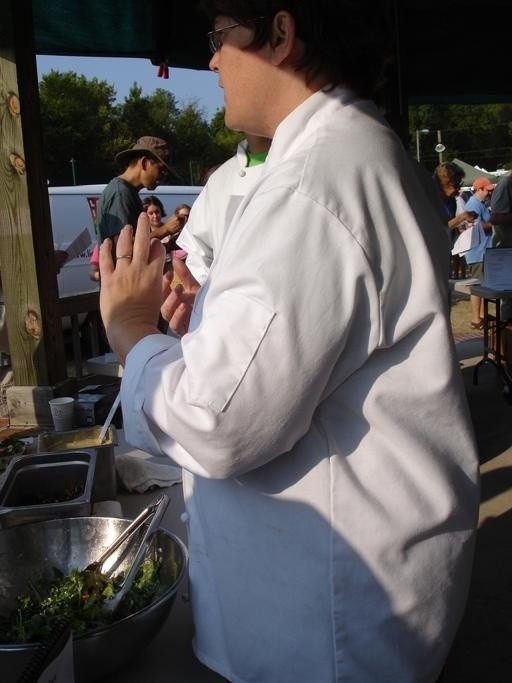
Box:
[118,256,131,258]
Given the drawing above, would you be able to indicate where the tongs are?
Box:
[83,492,170,623]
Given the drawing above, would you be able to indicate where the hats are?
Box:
[472,175,495,189]
[116,136,180,175]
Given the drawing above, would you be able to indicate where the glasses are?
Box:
[204,14,270,51]
[449,182,464,187]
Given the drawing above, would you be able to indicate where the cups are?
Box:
[48,397,74,433]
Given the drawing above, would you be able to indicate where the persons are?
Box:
[141,195,191,253]
[96,135,192,277]
[90,243,100,281]
[429,160,512,407]
[88,1,486,682]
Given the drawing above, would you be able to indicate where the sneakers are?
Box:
[471,312,496,330]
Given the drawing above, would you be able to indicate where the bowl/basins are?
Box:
[3,519,190,677]
